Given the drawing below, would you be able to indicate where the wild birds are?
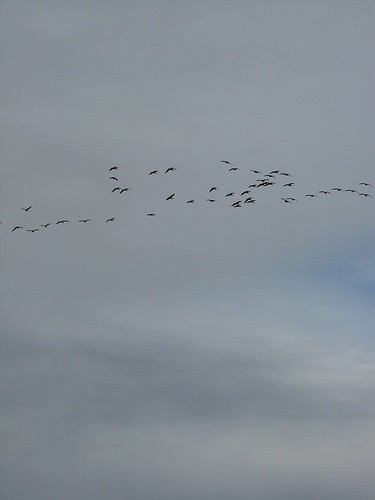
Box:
[78,219,90,222]
[120,188,128,194]
[112,187,119,192]
[360,182,371,187]
[21,206,32,211]
[165,167,176,173]
[109,167,117,171]
[149,171,158,175]
[40,223,51,227]
[147,214,155,216]
[56,220,69,224]
[187,200,195,203]
[221,160,293,189]
[166,193,175,200]
[110,177,119,181]
[206,186,255,208]
[12,226,23,232]
[27,229,39,232]
[106,217,116,223]
[280,188,371,203]
